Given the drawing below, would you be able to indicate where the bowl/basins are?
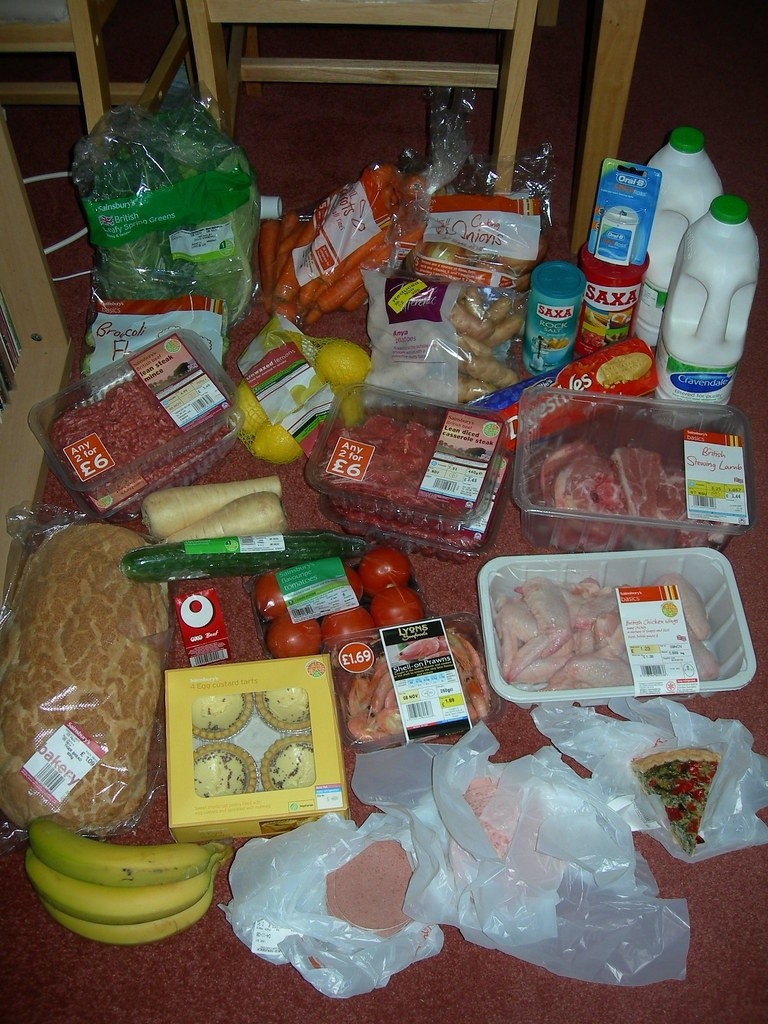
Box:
[511,386,757,553]
[44,408,245,522]
[28,330,240,499]
[306,382,511,523]
[478,547,757,707]
[317,450,511,564]
[321,612,506,750]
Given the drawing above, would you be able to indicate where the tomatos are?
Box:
[253,545,420,658]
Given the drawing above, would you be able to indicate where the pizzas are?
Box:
[631,747,721,855]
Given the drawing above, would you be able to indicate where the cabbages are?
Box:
[85,110,258,325]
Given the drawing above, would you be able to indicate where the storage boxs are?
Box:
[508,387,755,544]
[21,325,246,497]
[471,547,759,706]
[44,406,249,514]
[316,447,511,567]
[161,650,352,843]
[304,383,509,526]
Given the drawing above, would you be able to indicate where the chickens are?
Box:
[496,575,719,684]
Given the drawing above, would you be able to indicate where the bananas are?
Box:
[24,819,232,944]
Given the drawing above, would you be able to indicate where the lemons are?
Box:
[237,338,369,465]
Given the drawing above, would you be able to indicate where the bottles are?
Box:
[522,260,586,376]
[574,241,650,358]
[654,194,760,430]
[635,127,724,347]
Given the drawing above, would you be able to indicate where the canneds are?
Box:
[574,240,650,357]
[522,261,587,378]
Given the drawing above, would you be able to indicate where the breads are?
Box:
[0,523,168,835]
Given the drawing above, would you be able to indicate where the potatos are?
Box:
[448,287,526,400]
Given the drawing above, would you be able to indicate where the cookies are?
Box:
[595,352,652,388]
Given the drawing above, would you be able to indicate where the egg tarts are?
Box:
[191,686,315,797]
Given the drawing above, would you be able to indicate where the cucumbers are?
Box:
[120,528,370,583]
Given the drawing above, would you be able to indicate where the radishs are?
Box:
[139,474,289,543]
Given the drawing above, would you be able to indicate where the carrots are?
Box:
[260,163,429,322]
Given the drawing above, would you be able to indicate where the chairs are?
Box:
[0,0,197,143]
[182,0,541,196]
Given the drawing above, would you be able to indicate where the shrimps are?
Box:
[346,629,489,740]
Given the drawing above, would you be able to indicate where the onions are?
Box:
[416,231,550,291]
